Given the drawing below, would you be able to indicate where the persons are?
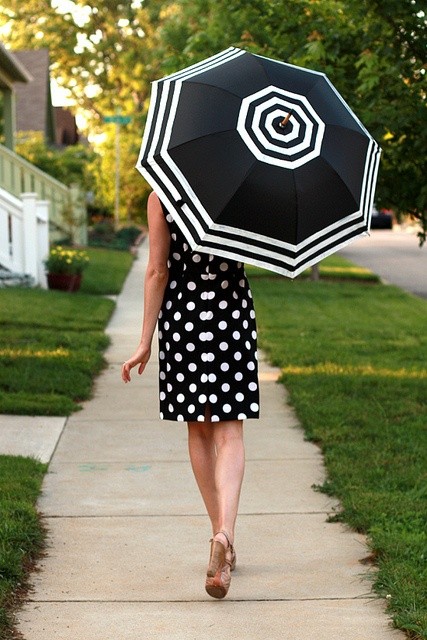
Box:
[121,191,260,599]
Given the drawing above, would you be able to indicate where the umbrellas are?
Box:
[136,46,383,283]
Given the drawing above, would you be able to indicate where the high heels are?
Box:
[205,531,235,599]
